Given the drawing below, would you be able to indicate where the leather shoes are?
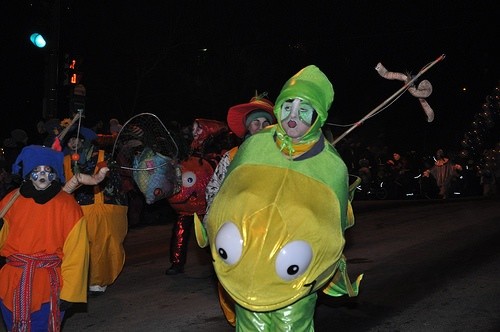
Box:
[165,263,184,274]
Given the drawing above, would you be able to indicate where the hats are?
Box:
[13,146,64,181]
[58,121,97,154]
[195,118,226,139]
[226,96,277,138]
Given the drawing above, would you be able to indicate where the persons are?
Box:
[192,64,364,332]
[51,124,129,292]
[202,89,277,327]
[165,118,233,276]
[0,84,500,227]
[0,144,90,332]
